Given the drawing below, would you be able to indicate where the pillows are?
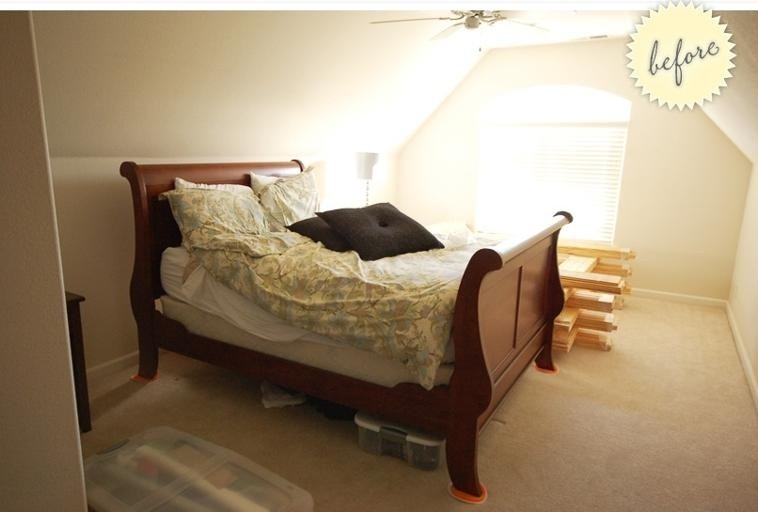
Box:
[315,203,445,261]
[259,166,325,231]
[282,216,353,252]
[174,176,256,198]
[249,171,285,193]
[158,189,271,240]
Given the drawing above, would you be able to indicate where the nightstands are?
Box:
[65,292,92,434]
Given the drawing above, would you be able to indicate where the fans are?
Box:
[367,11,552,44]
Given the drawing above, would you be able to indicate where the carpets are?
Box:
[86,424,314,512]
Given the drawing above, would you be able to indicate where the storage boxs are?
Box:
[353,409,447,472]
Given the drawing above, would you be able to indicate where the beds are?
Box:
[118,158,574,505]
